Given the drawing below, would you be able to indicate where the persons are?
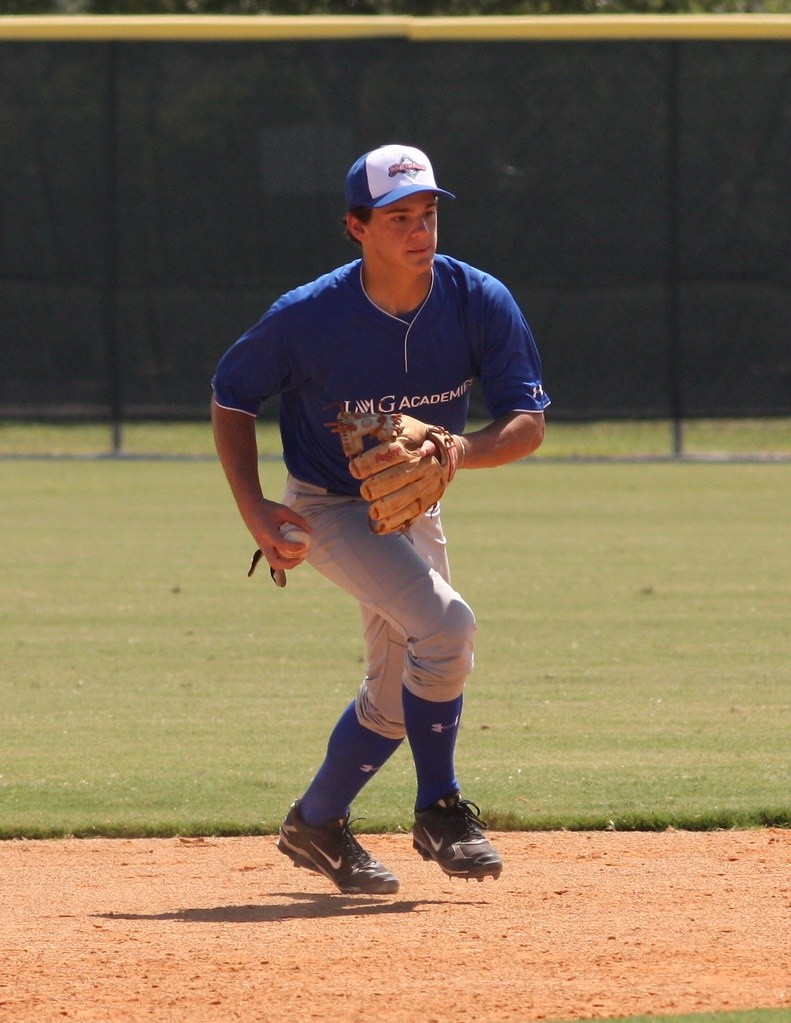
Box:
[212,144,550,896]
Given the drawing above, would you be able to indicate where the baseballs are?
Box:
[277,522,314,561]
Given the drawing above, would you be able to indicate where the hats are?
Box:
[345,144,455,208]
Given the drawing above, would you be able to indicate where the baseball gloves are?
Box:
[318,399,469,540]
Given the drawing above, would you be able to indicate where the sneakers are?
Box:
[276,799,400,894]
[412,789,502,883]
